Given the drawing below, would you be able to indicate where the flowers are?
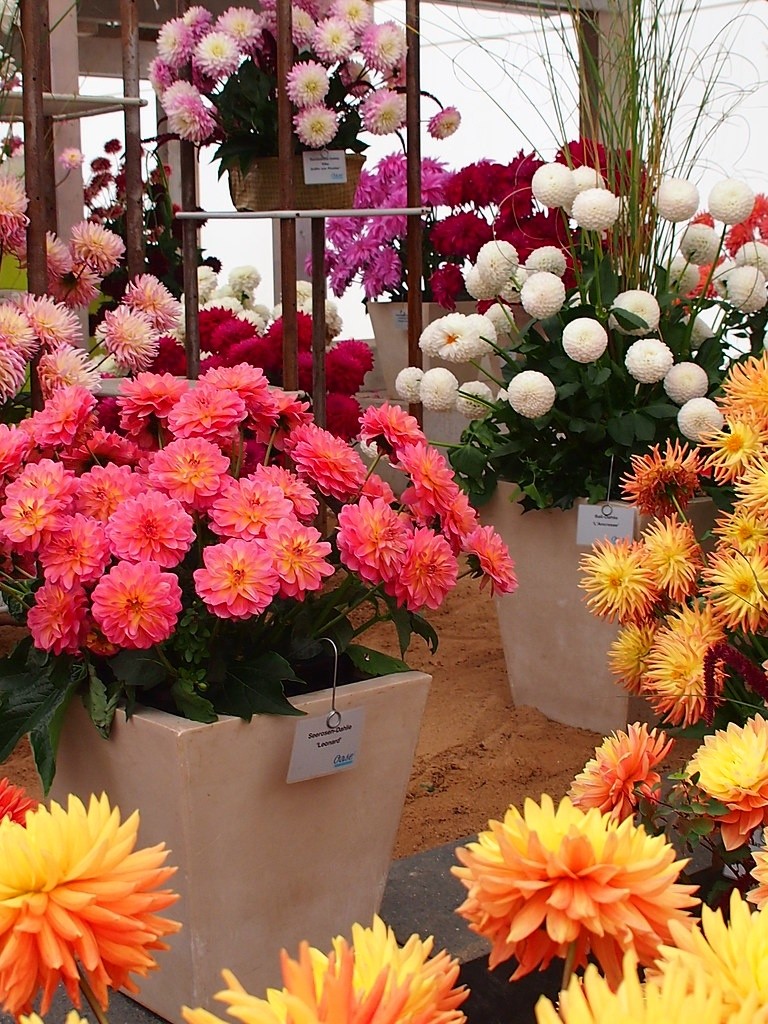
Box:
[425,140,659,316]
[571,352,768,725]
[394,157,767,510]
[72,126,220,286]
[306,152,454,302]
[160,262,342,353]
[1,364,520,793]
[671,189,767,332]
[1,169,184,414]
[0,725,767,1024]
[144,1,457,169]
[141,302,377,444]
[0,0,73,219]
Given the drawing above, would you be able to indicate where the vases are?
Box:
[365,292,552,464]
[226,147,369,212]
[466,471,723,736]
[36,642,434,1021]
